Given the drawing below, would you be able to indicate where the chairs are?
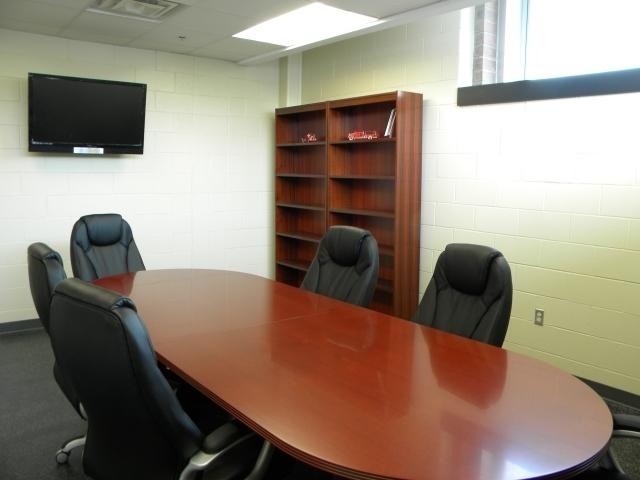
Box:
[46,276,274,480]
[608,409,640,480]
[298,225,380,307]
[69,212,143,280]
[27,240,87,464]
[412,242,513,348]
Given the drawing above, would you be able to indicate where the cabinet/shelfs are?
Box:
[275,92,423,321]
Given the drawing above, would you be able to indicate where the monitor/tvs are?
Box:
[28,72,147,155]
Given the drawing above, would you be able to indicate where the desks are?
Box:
[86,268,614,480]
